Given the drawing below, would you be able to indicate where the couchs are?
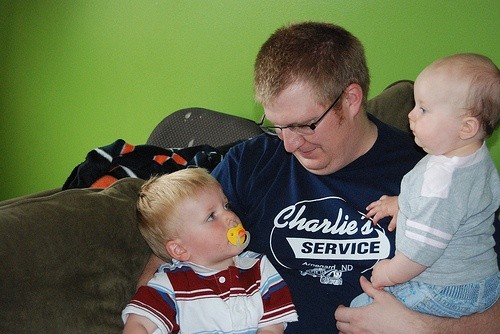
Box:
[0,78,423,334]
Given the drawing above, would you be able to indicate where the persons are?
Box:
[121,168,299,334]
[135,21,499,334]
[350,53,500,318]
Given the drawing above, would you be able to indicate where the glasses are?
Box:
[258,87,346,135]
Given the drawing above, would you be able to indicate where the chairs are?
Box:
[143,108,262,150]
